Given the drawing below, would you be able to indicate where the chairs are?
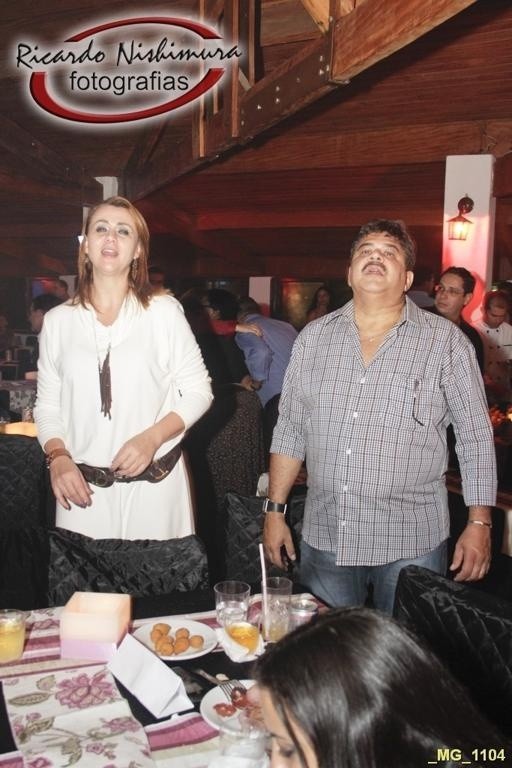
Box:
[0,328,511,768]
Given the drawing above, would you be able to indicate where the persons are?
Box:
[259,215,498,615]
[252,603,512,767]
[32,194,215,618]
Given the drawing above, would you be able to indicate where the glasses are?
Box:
[434,284,467,297]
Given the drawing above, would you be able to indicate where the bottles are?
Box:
[5,346,18,361]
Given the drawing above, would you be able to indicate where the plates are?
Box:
[129,616,225,664]
[198,679,275,737]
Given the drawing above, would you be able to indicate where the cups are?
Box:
[21,409,32,421]
[1,609,27,666]
[214,578,291,646]
[1,414,11,424]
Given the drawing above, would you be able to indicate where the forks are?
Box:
[173,661,245,700]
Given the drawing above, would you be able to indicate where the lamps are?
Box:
[446,194,475,241]
